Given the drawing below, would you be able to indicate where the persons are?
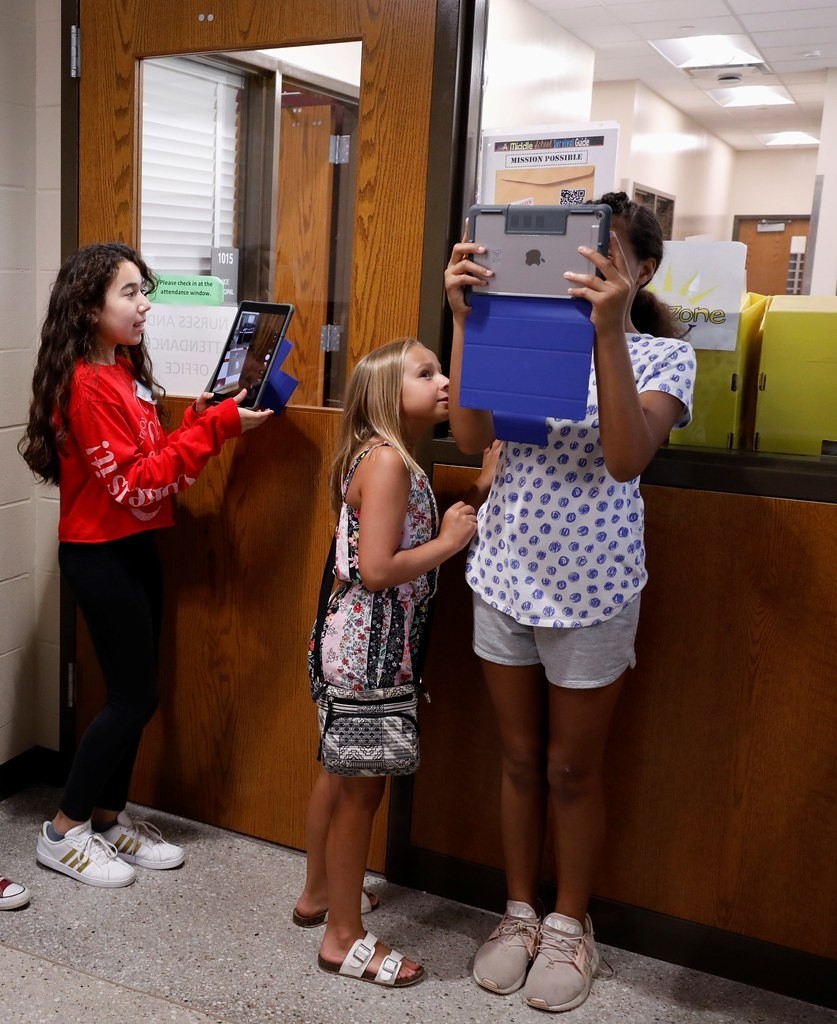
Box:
[37,242,273,887]
[445,192,696,1012]
[294,339,503,987]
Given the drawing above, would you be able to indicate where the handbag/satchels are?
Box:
[310,681,431,778]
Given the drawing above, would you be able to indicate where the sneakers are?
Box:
[36,818,136,888]
[0,875,31,911]
[523,912,615,1012]
[92,808,184,869]
[473,897,546,995]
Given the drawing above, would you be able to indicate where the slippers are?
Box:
[318,929,426,988]
[292,887,380,928]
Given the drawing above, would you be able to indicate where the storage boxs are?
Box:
[668,292,836,456]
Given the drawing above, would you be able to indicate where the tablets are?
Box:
[204,300,294,411]
[467,204,612,300]
